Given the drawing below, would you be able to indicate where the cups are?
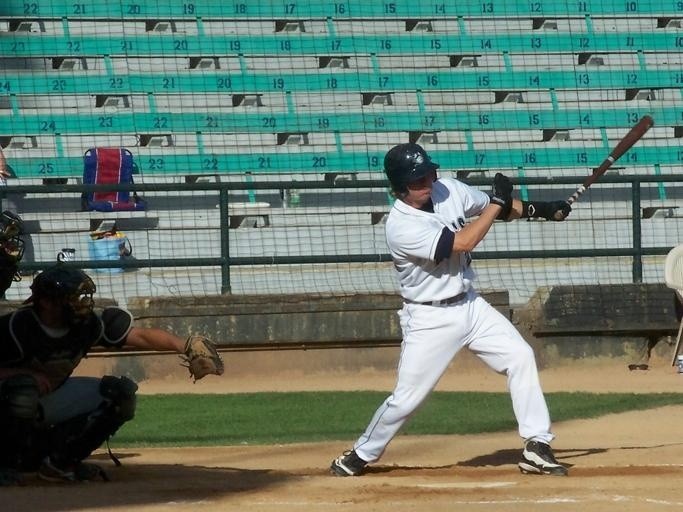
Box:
[677,354,683,372]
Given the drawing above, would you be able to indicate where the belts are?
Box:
[399,293,466,309]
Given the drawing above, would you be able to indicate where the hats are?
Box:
[383,141,438,184]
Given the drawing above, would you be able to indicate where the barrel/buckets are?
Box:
[88,237,128,273]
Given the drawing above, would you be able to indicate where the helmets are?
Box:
[23,265,96,304]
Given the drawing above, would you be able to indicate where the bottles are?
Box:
[287,179,300,207]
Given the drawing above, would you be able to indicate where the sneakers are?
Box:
[330,450,368,478]
[38,456,98,486]
[1,461,39,487]
[517,440,568,477]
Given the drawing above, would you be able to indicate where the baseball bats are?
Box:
[554,116,654,221]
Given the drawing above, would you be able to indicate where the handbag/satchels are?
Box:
[88,236,136,273]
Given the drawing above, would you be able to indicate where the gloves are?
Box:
[521,198,571,222]
[490,172,513,204]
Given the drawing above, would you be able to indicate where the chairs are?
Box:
[664,243,683,367]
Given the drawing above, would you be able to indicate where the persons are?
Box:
[329,141,572,478]
[0,263,229,485]
[0,145,11,180]
[0,210,25,300]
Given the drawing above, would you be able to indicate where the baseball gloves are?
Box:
[184,336,223,380]
